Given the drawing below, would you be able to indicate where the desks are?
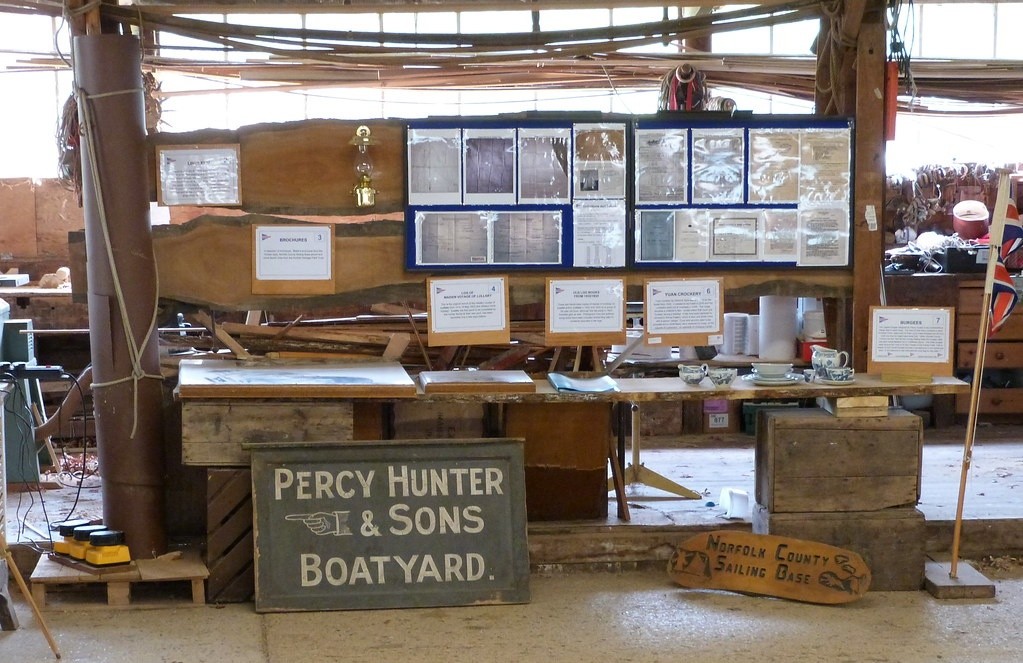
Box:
[174,355,970,519]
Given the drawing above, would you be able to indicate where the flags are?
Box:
[985,197,1023,337]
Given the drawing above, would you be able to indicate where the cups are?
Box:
[804,369,816,383]
[826,367,854,381]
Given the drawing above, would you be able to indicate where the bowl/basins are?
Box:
[751,363,793,377]
[708,369,738,387]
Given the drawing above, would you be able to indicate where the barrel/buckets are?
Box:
[719,489,748,520]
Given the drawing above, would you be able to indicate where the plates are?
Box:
[742,374,804,386]
[820,378,856,386]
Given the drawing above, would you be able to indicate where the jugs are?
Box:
[677,362,708,386]
[812,345,849,379]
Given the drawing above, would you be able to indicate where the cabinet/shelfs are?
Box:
[952,279,1023,427]
